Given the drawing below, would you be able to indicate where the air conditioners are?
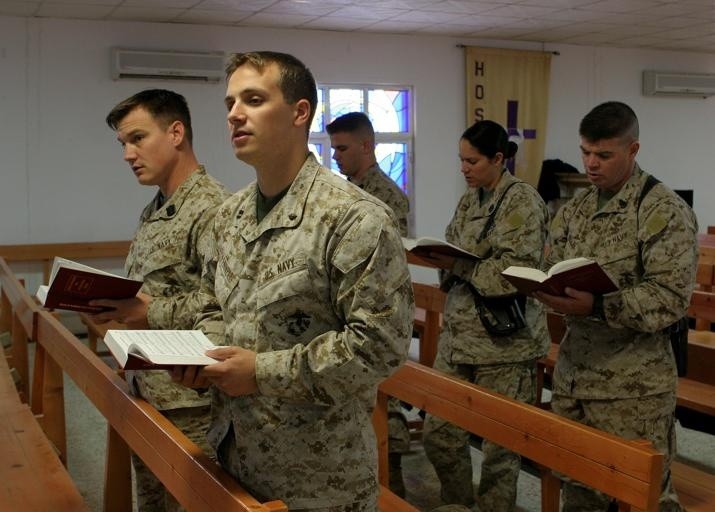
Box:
[110,46,226,84]
[643,69,715,99]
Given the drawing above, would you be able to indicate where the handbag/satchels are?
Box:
[668,317,687,378]
[473,292,526,339]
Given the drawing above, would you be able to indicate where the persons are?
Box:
[168,50,417,512]
[534,98,699,512]
[325,110,411,499]
[106,88,234,512]
[420,118,554,512]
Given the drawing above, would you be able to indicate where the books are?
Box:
[500,256,619,301]
[36,255,144,314]
[102,328,224,371]
[400,236,481,263]
